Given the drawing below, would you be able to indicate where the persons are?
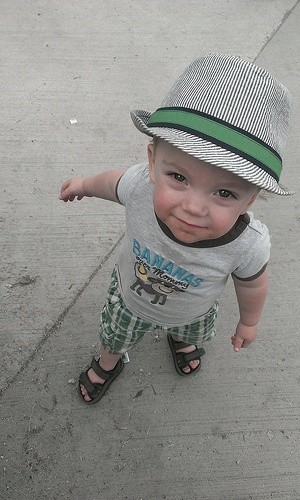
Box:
[58,54,298,406]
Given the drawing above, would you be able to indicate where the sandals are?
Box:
[78,355,125,404]
[167,333,206,377]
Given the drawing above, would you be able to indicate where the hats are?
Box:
[129,52,296,197]
